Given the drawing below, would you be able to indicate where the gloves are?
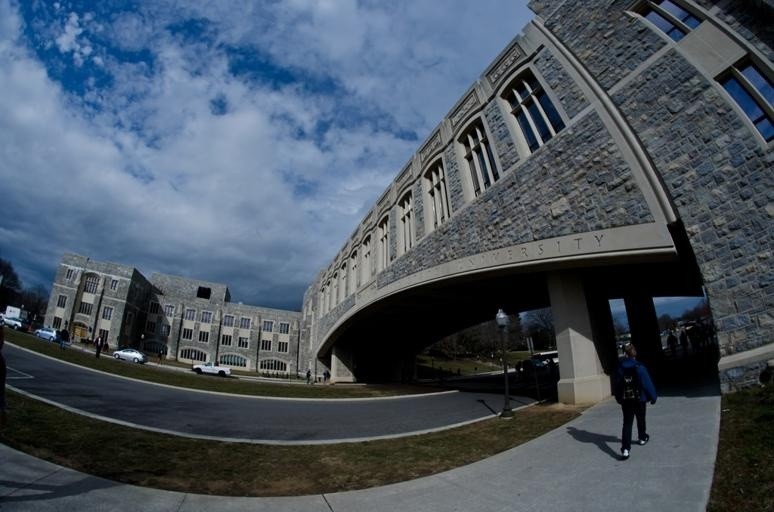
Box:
[650,399,656,404]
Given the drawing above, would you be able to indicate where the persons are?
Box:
[667,319,716,359]
[59,326,68,349]
[615,344,658,459]
[96,335,103,358]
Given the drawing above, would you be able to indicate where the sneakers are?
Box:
[620,447,630,460]
[639,434,649,445]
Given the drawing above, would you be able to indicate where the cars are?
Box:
[0,313,29,332]
[34,327,60,342]
[514,349,561,384]
[112,348,148,365]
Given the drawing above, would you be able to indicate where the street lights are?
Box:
[495,307,517,420]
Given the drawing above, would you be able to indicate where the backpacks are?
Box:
[613,365,644,406]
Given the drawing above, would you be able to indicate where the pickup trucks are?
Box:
[192,361,232,379]
